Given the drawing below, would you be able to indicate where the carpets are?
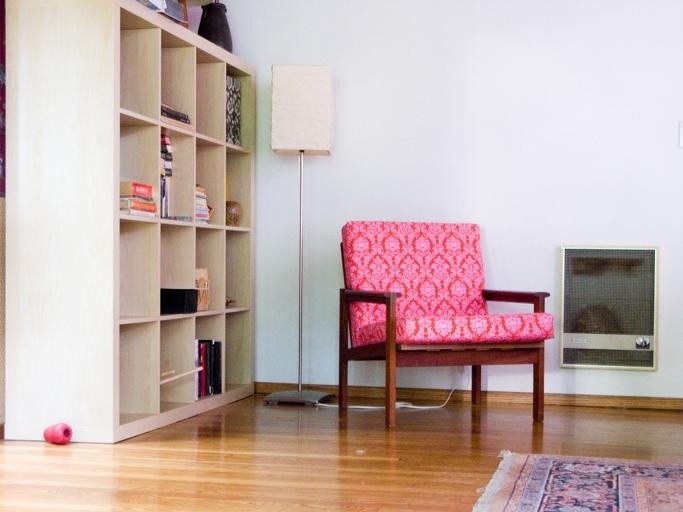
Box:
[472,454,683,511]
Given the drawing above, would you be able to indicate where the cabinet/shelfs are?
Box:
[6,0,258,445]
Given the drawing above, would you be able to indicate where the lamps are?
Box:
[264,66,331,405]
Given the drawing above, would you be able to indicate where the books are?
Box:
[159,133,173,220]
[161,103,194,131]
[120,180,159,220]
[195,338,223,402]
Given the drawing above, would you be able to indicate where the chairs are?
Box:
[337,219,554,429]
